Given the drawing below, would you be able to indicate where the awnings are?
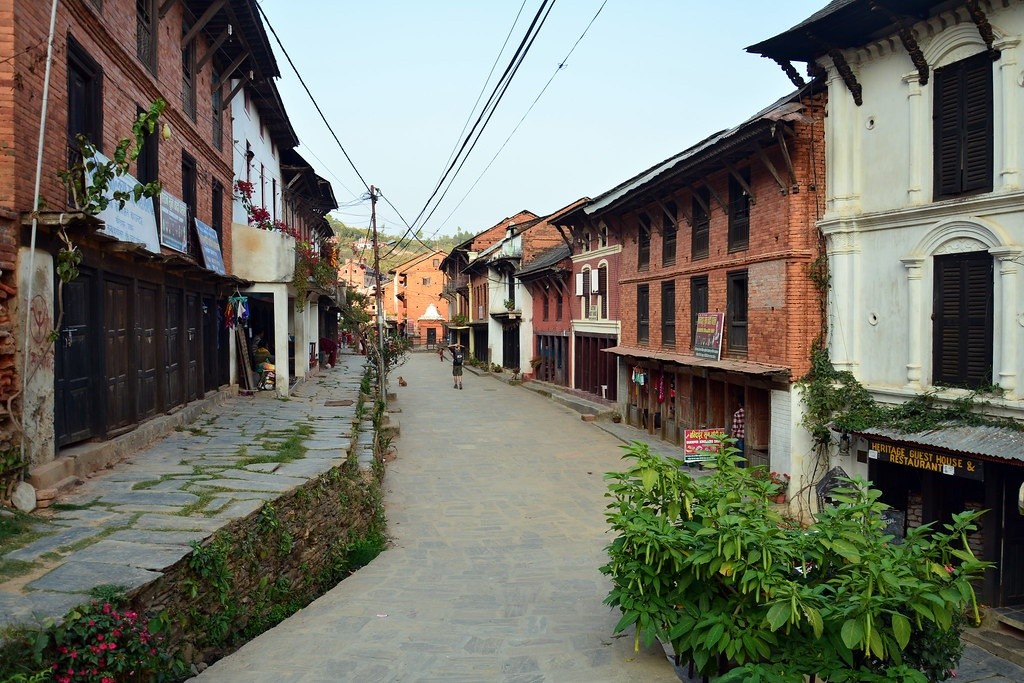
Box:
[600,346,789,377]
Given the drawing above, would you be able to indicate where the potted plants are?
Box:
[504,299,514,311]
[613,413,621,423]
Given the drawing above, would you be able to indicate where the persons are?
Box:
[342,334,346,348]
[731,399,744,468]
[439,348,444,362]
[452,347,464,390]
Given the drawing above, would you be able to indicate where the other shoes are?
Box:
[454,384,458,389]
[459,382,462,389]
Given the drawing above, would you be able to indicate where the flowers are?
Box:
[236,178,338,313]
[769,472,790,493]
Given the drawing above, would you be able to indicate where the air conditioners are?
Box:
[591,268,598,293]
[575,273,583,296]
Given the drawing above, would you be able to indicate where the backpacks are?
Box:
[454,350,463,364]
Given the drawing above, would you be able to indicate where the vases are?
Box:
[776,493,786,504]
[967,610,986,628]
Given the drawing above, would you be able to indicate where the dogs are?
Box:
[397,376,407,387]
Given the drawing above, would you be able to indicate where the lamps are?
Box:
[839,433,850,455]
